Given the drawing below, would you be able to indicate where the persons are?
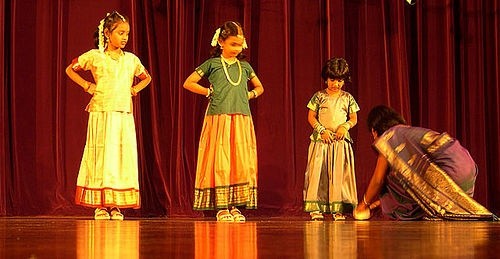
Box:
[183,21,264,220]
[303,57,360,220]
[65,11,152,221]
[353,105,478,220]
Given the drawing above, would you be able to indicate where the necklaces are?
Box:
[222,57,242,85]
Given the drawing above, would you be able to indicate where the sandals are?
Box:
[230,208,245,222]
[333,213,345,221]
[94,208,110,220]
[310,210,324,221]
[109,207,124,220]
[216,209,234,222]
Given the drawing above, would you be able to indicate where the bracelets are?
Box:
[252,90,257,98]
[373,203,376,207]
[132,87,137,96]
[342,123,350,130]
[313,122,325,134]
[206,88,210,98]
[364,195,369,204]
[347,121,353,128]
[85,82,91,92]
